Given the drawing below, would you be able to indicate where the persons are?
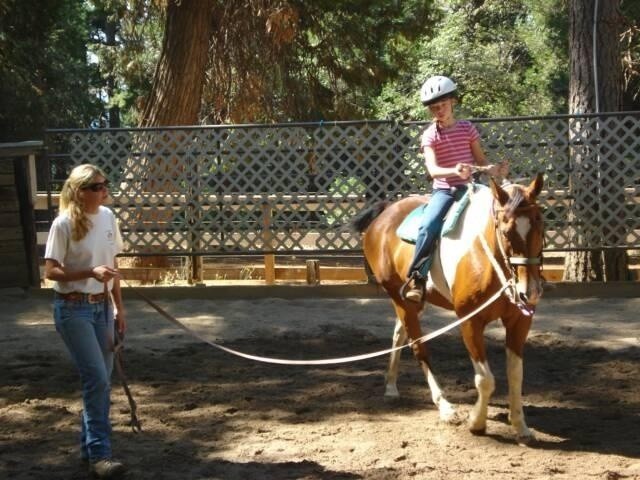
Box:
[404,75,556,302]
[45,164,127,478]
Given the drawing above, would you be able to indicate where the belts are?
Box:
[54,291,107,304]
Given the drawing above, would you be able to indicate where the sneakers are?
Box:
[89,459,128,478]
[405,283,424,302]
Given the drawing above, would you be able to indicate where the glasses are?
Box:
[81,179,110,192]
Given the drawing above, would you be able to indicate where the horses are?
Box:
[345,173,545,447]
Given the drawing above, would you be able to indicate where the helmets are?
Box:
[420,76,458,106]
